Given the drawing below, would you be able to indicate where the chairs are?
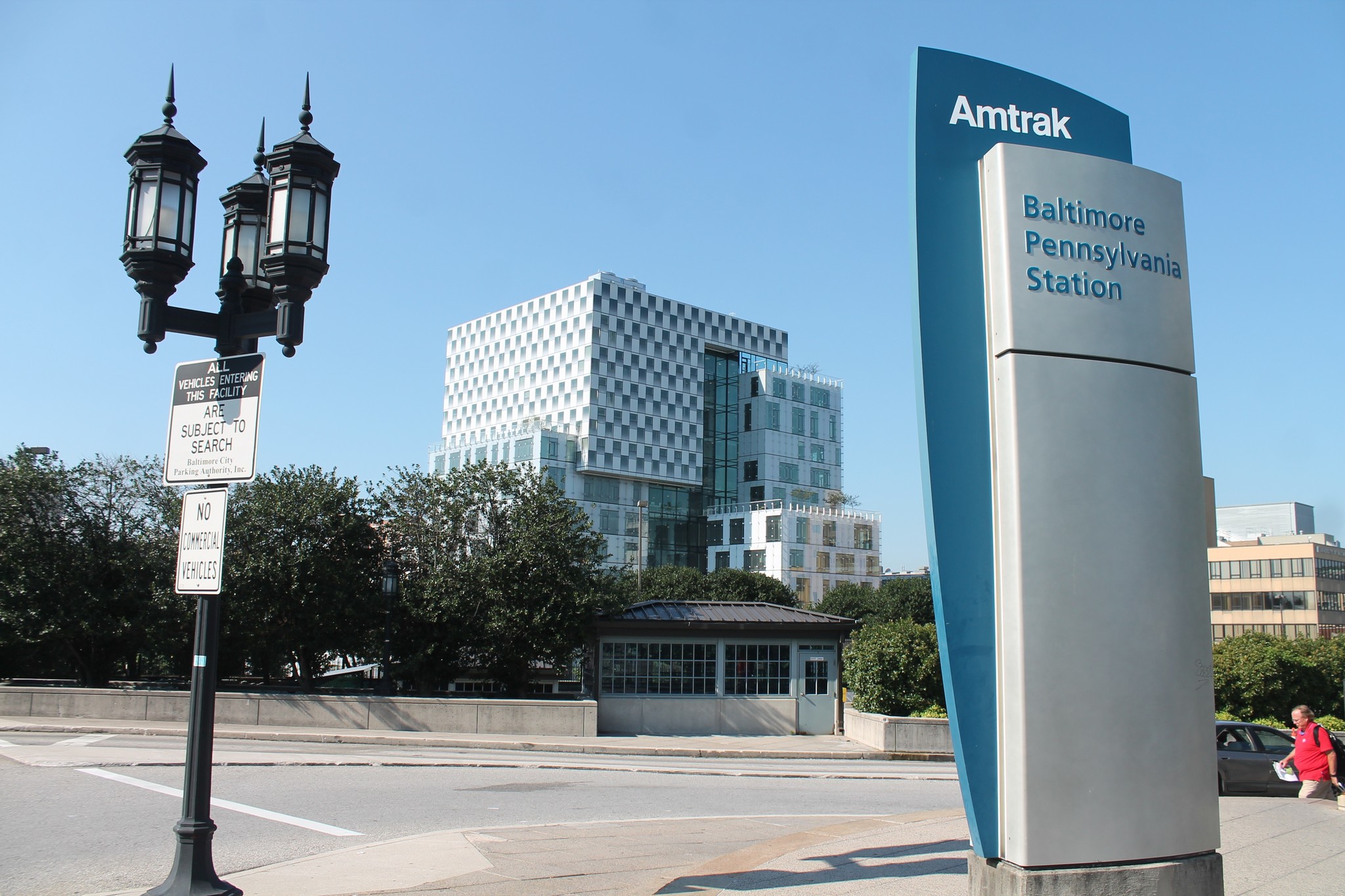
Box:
[1228,741,1242,751]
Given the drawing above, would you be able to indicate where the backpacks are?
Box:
[1313,725,1345,797]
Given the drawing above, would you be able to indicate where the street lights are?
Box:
[116,61,346,896]
[380,546,402,696]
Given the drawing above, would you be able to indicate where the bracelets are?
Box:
[1330,774,1337,777]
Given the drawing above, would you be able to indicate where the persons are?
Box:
[1217,731,1229,748]
[1280,705,1340,801]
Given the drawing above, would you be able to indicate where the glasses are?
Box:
[1292,717,1303,722]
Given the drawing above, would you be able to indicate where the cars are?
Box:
[1214,720,1303,798]
